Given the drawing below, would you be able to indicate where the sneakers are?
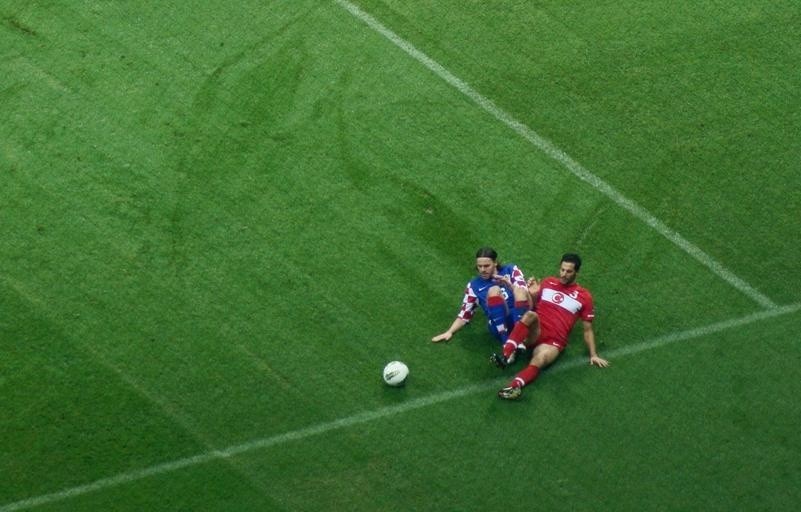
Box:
[518,341,528,354]
[489,354,508,370]
[498,386,523,400]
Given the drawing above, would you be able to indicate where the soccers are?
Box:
[384,361,410,386]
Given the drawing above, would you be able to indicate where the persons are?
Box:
[488,252,610,402]
[432,245,537,366]
